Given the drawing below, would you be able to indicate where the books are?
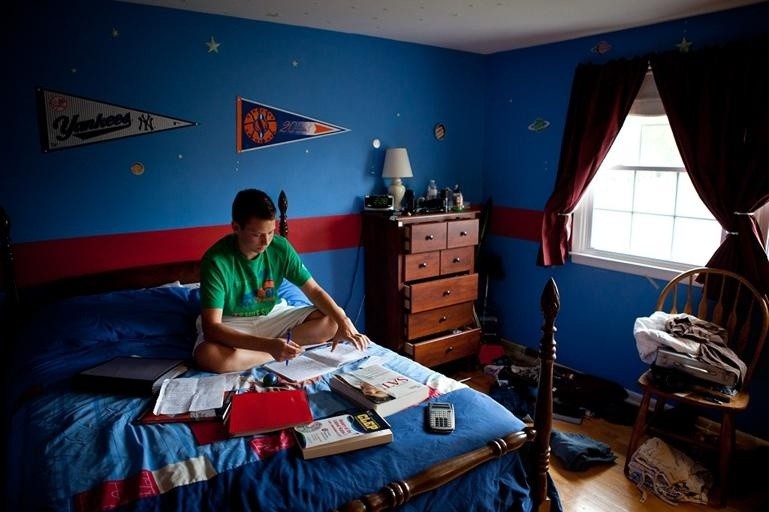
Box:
[293,407,395,464]
[329,361,442,416]
[229,388,312,438]
[79,353,187,398]
[155,375,229,417]
[263,338,369,384]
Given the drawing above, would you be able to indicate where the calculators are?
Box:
[428,402,455,431]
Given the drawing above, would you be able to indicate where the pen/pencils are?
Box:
[286,328,291,366]
[218,391,235,426]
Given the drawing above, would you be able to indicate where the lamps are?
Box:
[381,148,414,210]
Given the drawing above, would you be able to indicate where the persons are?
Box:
[191,187,372,374]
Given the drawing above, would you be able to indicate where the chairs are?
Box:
[624,267,769,505]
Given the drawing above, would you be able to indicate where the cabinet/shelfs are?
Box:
[359,208,482,368]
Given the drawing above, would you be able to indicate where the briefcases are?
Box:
[655,349,736,386]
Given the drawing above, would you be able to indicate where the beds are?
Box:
[0,189,562,509]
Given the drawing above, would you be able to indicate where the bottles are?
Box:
[428,180,438,200]
